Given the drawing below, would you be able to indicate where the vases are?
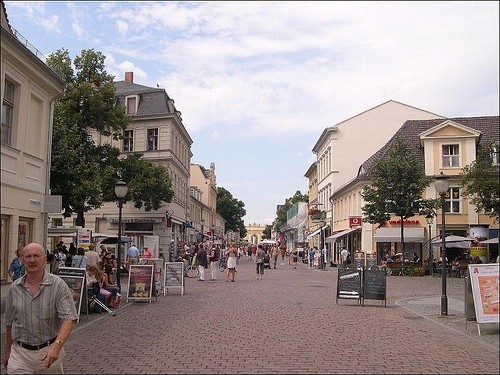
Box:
[413,271,424,275]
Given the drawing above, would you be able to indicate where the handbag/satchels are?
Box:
[92,282,100,294]
[115,292,122,308]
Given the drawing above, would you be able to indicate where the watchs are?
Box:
[54,340,63,346]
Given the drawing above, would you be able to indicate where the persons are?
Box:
[3,243,78,374]
[439,255,483,278]
[7,237,419,313]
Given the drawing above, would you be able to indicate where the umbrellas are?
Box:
[100,236,132,244]
[78,233,108,238]
[430,235,499,244]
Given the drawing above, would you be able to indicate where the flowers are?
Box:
[414,267,424,272]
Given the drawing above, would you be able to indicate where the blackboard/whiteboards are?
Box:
[126,264,157,299]
[164,262,184,288]
[337,267,362,299]
[354,258,364,268]
[362,266,387,300]
[57,267,89,316]
[367,257,377,267]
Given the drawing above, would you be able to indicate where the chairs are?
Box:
[387,259,468,276]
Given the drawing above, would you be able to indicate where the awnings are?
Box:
[307,226,328,239]
[374,228,424,243]
[326,229,358,242]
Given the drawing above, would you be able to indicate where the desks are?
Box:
[139,258,163,278]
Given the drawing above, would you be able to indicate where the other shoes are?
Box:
[210,279,216,281]
[198,279,205,281]
[231,280,235,282]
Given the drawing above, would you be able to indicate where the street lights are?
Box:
[424,213,433,276]
[200,218,205,246]
[211,224,215,244]
[434,171,451,318]
[115,178,129,293]
[220,228,224,249]
[318,220,325,271]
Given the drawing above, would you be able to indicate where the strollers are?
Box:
[262,254,271,269]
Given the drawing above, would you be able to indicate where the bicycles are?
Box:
[185,250,199,278]
[217,256,228,272]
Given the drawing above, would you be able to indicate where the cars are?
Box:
[91,232,134,257]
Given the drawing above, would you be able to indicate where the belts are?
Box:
[15,336,57,351]
